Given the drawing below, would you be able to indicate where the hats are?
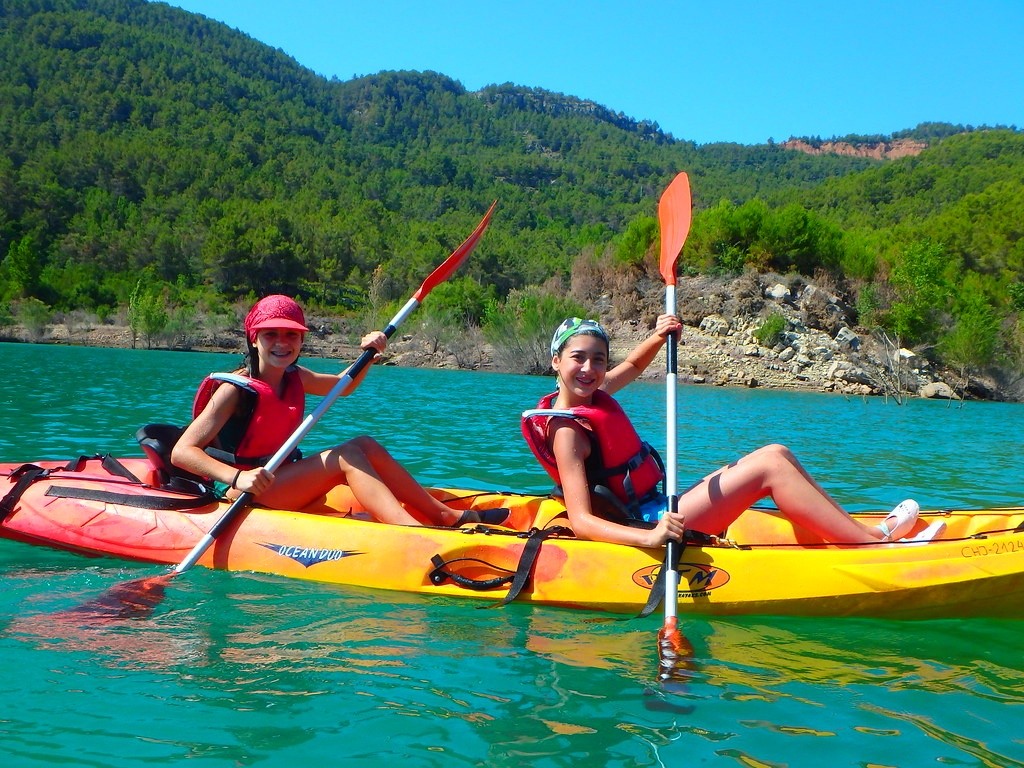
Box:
[247,295,308,343]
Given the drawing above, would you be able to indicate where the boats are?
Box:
[0,459,1024,615]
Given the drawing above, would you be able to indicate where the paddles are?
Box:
[650,169,696,683]
[82,194,501,620]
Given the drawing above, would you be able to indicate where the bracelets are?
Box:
[232,468,246,489]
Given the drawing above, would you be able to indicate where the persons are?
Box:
[171,294,513,529]
[545,314,946,549]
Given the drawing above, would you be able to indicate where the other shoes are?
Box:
[452,507,511,528]
[899,519,947,546]
[876,499,920,542]
